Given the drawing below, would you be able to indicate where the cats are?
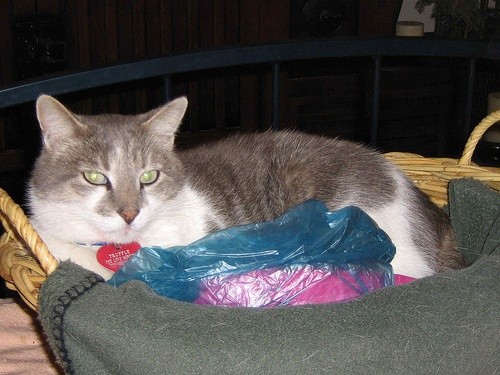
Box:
[21,93,461,284]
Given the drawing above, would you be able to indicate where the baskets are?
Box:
[0,105,500,314]
